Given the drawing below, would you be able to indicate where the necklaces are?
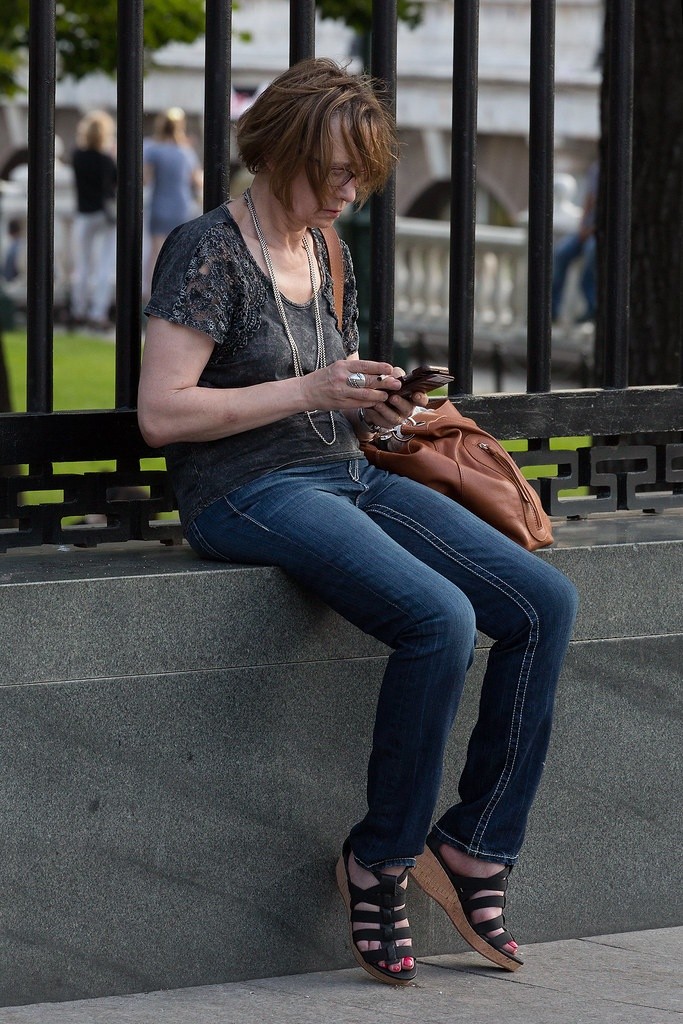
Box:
[242,188,340,448]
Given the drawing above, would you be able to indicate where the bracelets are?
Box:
[358,408,395,435]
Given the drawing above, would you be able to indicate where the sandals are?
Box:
[409,832,523,973]
[335,836,418,985]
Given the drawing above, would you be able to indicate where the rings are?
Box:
[345,372,370,391]
[391,417,403,425]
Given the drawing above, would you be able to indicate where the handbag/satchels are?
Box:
[359,397,554,552]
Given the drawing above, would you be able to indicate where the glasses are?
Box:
[310,164,370,187]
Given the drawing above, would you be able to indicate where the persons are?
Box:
[140,107,204,292]
[137,56,578,986]
[546,134,607,328]
[56,109,119,331]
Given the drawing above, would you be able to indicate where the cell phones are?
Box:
[379,367,454,405]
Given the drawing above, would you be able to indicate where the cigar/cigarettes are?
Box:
[377,374,389,382]
